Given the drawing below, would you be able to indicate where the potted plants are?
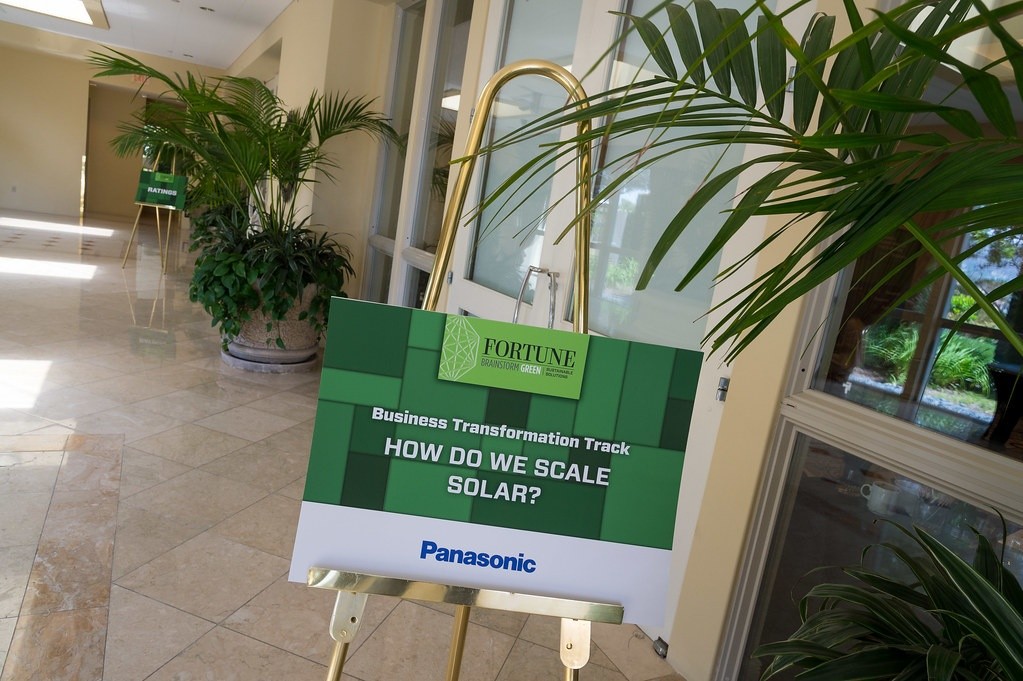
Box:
[84,43,406,373]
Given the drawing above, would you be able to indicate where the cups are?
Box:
[861,482,901,517]
